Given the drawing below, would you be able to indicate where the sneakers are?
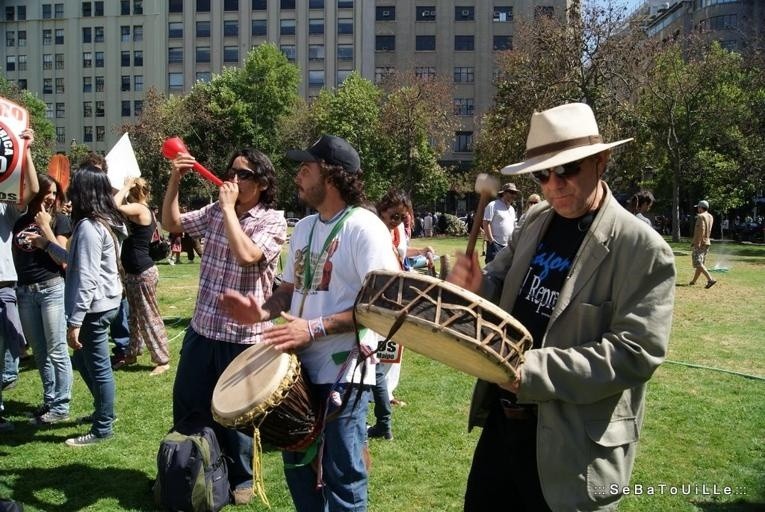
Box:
[0,419,14,429]
[22,403,50,418]
[232,486,253,505]
[75,414,119,425]
[367,425,392,439]
[29,411,71,425]
[705,280,716,289]
[65,431,114,447]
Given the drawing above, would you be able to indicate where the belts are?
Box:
[500,398,538,420]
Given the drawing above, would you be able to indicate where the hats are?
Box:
[693,200,709,209]
[498,183,520,194]
[500,102,634,175]
[287,133,360,175]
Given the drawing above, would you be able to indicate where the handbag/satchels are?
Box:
[148,206,170,261]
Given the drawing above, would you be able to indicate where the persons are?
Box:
[217,133,402,512]
[0,124,205,447]
[409,188,765,245]
[444,103,678,512]
[688,200,717,289]
[367,362,394,440]
[161,146,288,505]
[483,182,520,262]
[372,189,434,408]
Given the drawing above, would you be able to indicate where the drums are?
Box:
[354,269,533,386]
[212,341,319,450]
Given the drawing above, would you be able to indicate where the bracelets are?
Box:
[309,316,327,342]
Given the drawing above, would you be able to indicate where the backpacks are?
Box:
[151,414,235,512]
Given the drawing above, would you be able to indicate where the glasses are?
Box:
[393,213,407,220]
[505,190,517,194]
[225,167,255,180]
[529,157,588,185]
[529,201,538,205]
